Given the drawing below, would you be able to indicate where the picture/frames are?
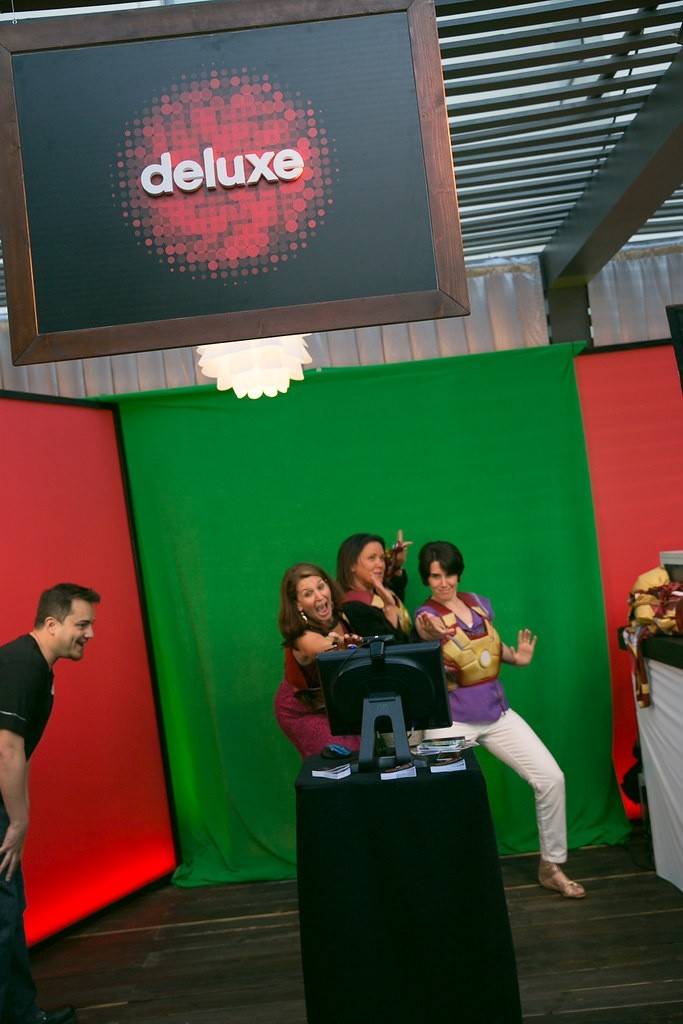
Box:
[0,0,473,366]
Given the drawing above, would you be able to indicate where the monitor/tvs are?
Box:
[315,642,454,773]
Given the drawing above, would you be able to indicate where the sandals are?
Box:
[537,859,586,898]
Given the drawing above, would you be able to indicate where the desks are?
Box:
[293,735,520,1024]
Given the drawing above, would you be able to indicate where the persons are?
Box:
[276,531,592,900]
[0,582,170,1024]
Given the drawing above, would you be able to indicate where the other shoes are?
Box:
[39,1005,79,1024]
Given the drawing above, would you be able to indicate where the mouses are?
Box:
[321,744,353,759]
